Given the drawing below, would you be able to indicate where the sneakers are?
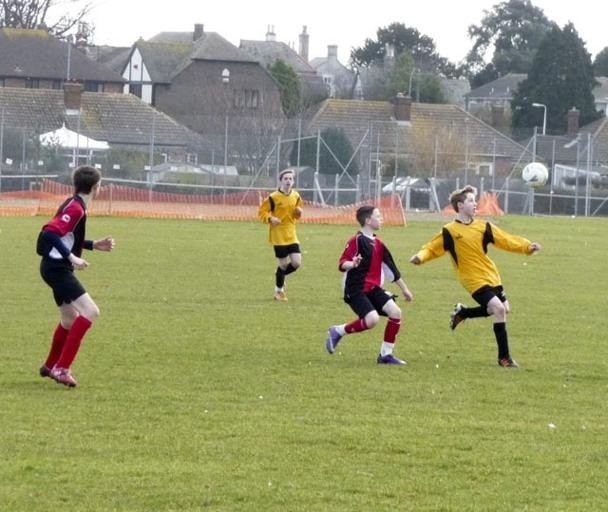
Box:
[274,291,288,302]
[326,326,342,353]
[450,303,464,330]
[39,365,77,387]
[377,354,407,365]
[499,358,518,367]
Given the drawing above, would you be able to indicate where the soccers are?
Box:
[522,162,548,186]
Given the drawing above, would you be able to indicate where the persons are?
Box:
[409,185,541,369]
[257,169,305,302]
[36,165,116,387]
[326,205,412,366]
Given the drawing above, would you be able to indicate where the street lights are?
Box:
[532,103,547,134]
